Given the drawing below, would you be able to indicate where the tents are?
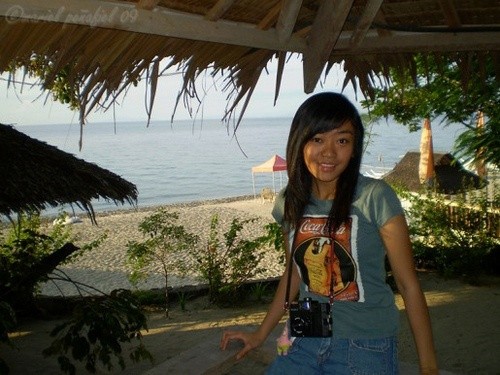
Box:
[251,153,288,201]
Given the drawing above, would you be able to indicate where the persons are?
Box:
[220,92,438,375]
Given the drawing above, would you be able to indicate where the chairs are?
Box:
[260,188,275,203]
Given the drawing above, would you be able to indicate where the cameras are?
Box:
[289,297,332,338]
